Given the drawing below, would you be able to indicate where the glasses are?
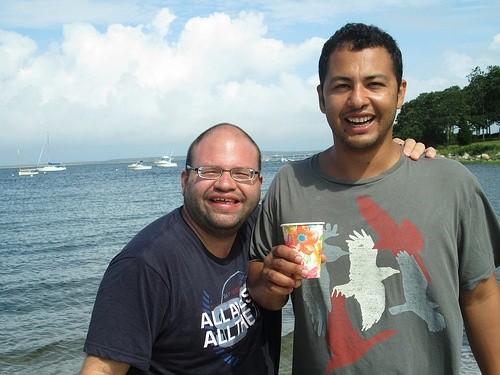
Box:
[186,164,261,181]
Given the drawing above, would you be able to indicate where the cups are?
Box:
[280,222,325,280]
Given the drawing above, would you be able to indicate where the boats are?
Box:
[128,161,153,170]
[18,147,40,176]
[261,152,296,162]
[36,142,66,172]
[154,152,178,167]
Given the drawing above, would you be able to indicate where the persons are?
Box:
[78,123,281,375]
[247,22,499,374]
